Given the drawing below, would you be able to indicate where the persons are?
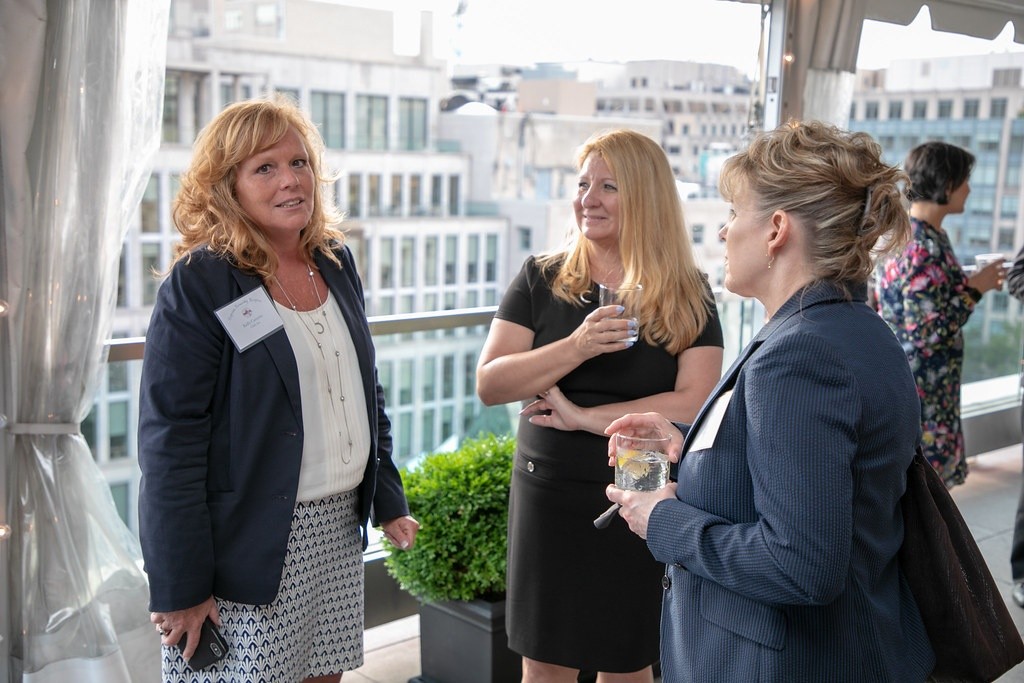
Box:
[476,128,724,683]
[136,99,421,683]
[1008,243,1024,607]
[605,121,936,683]
[868,142,1007,491]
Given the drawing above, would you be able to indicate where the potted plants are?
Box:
[375,429,661,683]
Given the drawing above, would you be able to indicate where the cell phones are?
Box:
[177,614,229,671]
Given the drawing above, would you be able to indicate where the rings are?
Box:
[159,627,168,636]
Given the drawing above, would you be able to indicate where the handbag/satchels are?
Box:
[898,446,1024,683]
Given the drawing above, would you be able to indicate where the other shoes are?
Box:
[1012,579,1024,606]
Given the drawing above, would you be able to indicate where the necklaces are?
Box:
[274,263,354,464]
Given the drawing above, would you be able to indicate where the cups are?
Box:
[975,253,1003,284]
[599,282,643,342]
[614,428,673,494]
